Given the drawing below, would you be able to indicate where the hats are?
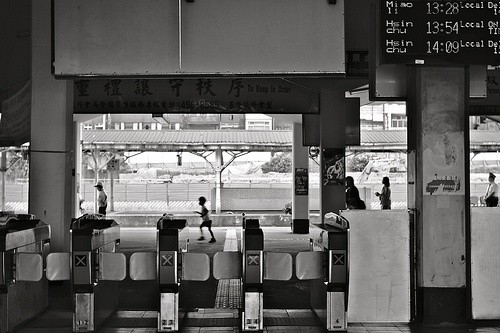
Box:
[489,172,496,179]
[94,184,103,188]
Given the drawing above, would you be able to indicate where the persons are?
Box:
[94,181,108,215]
[194,196,217,243]
[344,176,360,210]
[484,171,499,207]
[374,176,391,210]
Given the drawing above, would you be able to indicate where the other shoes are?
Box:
[208,238,216,243]
[198,237,205,241]
